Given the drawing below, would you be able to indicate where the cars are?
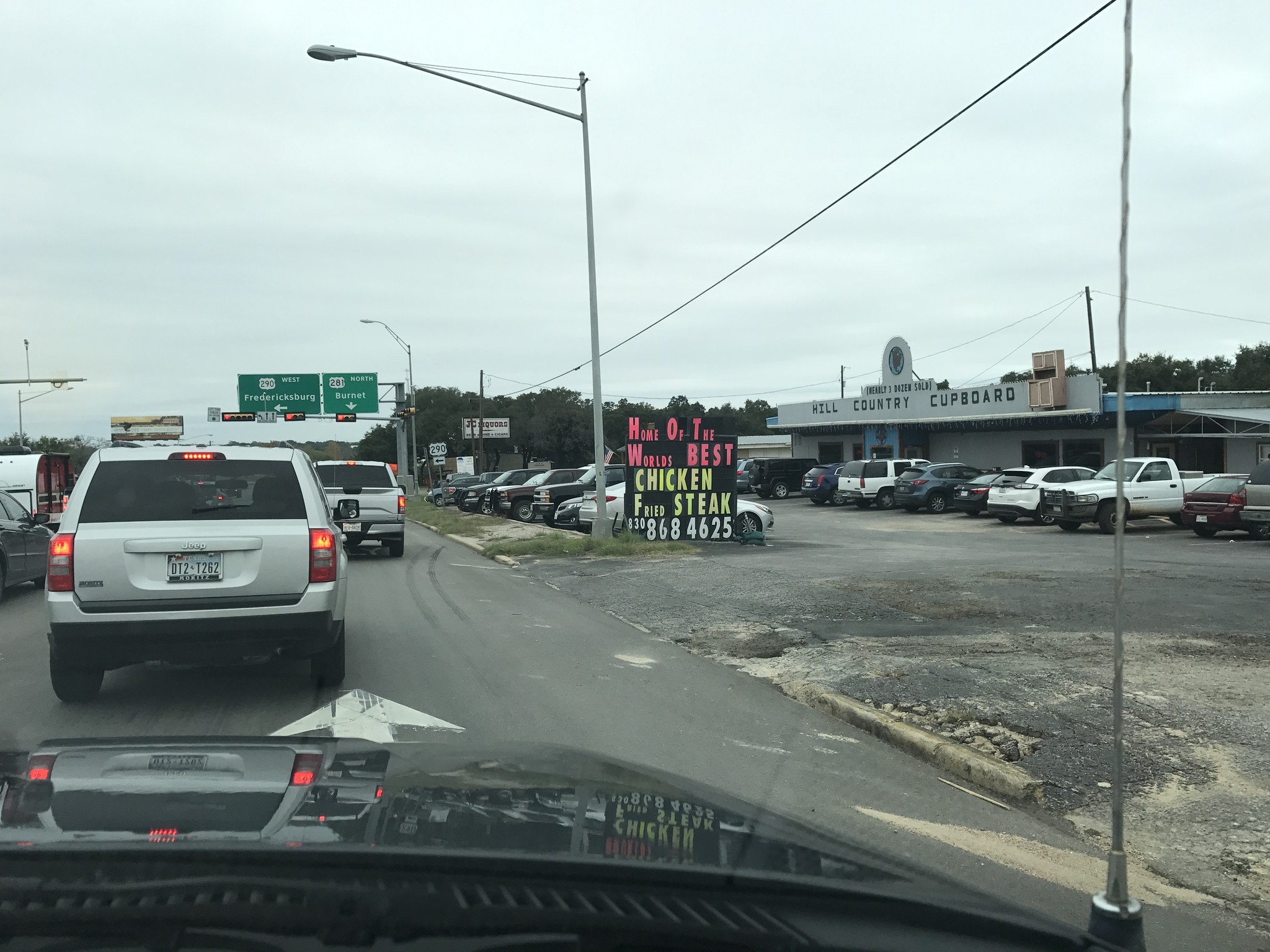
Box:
[425,476,479,507]
[578,481,775,541]
[1181,474,1251,536]
[0,490,57,598]
[554,497,591,534]
[954,471,1004,516]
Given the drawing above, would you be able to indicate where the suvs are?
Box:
[529,463,626,529]
[485,474,545,518]
[894,462,984,513]
[987,465,1111,525]
[44,443,346,701]
[1240,453,1270,541]
[312,460,406,559]
[446,472,470,483]
[748,456,819,499]
[456,468,549,516]
[736,457,784,492]
[489,467,588,524]
[800,461,856,505]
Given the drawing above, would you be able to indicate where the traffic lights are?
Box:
[335,413,356,423]
[284,412,305,421]
[397,406,416,419]
[221,411,256,422]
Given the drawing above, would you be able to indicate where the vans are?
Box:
[838,458,932,510]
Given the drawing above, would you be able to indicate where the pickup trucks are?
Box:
[442,472,506,508]
[1038,456,1251,535]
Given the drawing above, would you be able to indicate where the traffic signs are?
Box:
[237,373,322,415]
[322,372,379,415]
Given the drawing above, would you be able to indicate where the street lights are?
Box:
[361,319,420,498]
[307,46,618,546]
[19,387,75,453]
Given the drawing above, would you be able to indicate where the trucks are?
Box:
[0,444,74,533]
